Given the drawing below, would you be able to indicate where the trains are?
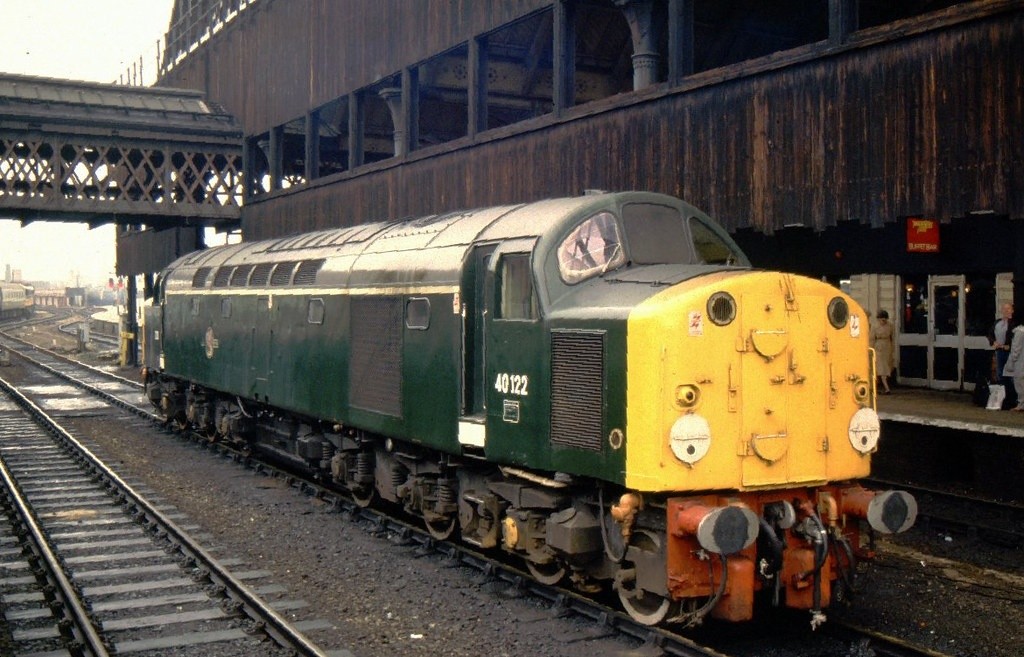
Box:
[145,190,919,634]
[0,282,38,322]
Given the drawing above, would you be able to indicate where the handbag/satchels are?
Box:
[986,385,1006,410]
[887,367,897,387]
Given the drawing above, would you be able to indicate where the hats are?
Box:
[876,310,888,318]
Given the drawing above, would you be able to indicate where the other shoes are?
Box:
[882,391,891,395]
[1010,405,1024,411]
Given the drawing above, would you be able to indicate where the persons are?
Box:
[984,301,1015,410]
[868,308,898,395]
[1001,309,1024,410]
